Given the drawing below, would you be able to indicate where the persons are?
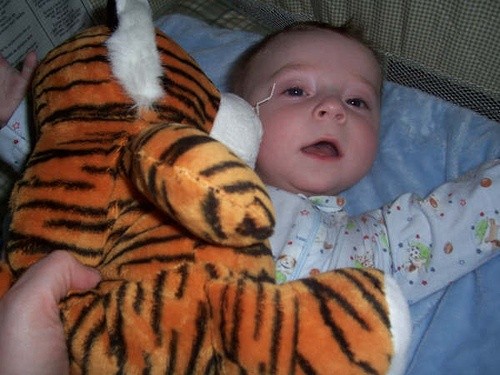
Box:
[0,250,102,374]
[1,20,500,307]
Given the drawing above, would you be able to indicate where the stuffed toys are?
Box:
[1,1,410,375]
